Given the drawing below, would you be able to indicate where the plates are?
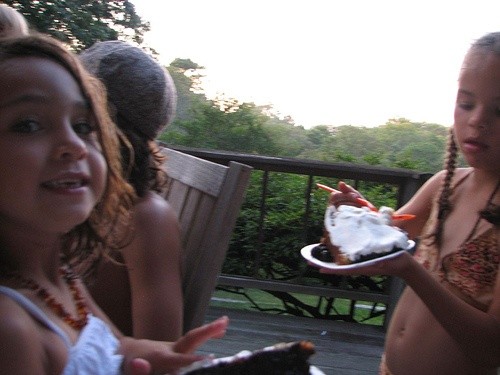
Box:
[300,240,415,270]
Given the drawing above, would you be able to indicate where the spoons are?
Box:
[315,182,416,222]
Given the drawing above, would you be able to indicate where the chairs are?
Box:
[146,139,254,336]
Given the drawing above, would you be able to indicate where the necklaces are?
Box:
[6,261,89,331]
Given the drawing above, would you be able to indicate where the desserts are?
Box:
[321,202,409,265]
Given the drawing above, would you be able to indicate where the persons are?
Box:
[0,36,227,375]
[1,5,28,38]
[330,30,499,375]
[72,38,184,343]
